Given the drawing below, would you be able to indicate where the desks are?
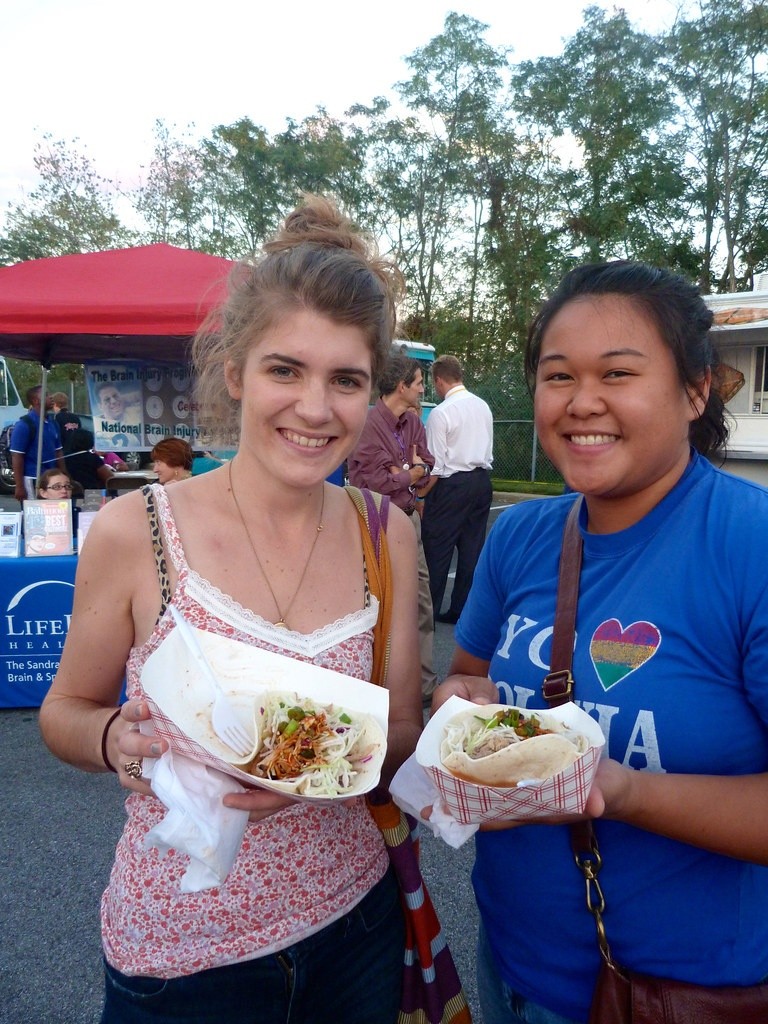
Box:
[106,477,156,489]
[0,537,129,706]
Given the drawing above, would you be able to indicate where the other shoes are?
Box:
[436,612,458,625]
[422,698,432,709]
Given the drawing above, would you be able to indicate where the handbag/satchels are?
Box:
[362,784,474,1024]
[589,959,768,1024]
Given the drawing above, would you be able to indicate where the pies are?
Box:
[441,703,589,785]
[192,688,387,796]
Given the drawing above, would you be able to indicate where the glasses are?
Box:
[47,485,74,490]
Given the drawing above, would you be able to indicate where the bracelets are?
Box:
[415,494,424,503]
[102,709,121,773]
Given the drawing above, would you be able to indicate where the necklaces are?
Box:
[229,458,325,631]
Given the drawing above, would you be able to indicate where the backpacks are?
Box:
[0,416,37,469]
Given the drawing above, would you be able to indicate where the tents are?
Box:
[0,242,262,499]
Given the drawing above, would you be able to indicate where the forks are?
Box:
[169,603,255,757]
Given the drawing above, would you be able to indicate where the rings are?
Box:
[124,761,142,776]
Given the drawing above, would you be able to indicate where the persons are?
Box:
[151,438,193,483]
[432,261,768,1024]
[40,193,424,1024]
[10,385,129,538]
[95,383,142,426]
[351,355,492,709]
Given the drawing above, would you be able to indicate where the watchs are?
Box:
[415,464,429,476]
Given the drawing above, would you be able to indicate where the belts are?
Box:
[404,507,415,516]
